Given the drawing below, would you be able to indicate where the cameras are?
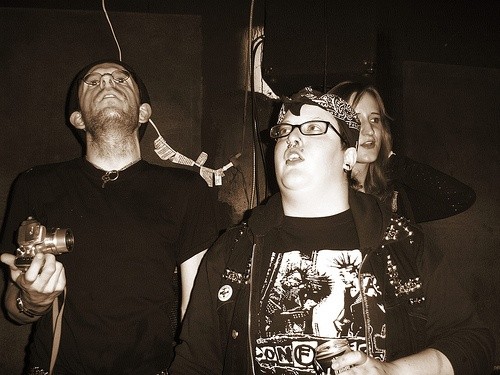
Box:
[15,217,74,275]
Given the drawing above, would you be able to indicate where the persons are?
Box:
[1,59,220,375]
[320,79,477,228]
[166,87,497,375]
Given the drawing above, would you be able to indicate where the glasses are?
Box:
[270,121,347,144]
[81,70,130,85]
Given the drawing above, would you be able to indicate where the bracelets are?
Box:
[16,290,54,319]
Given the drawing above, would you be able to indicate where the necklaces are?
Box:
[85,155,142,187]
